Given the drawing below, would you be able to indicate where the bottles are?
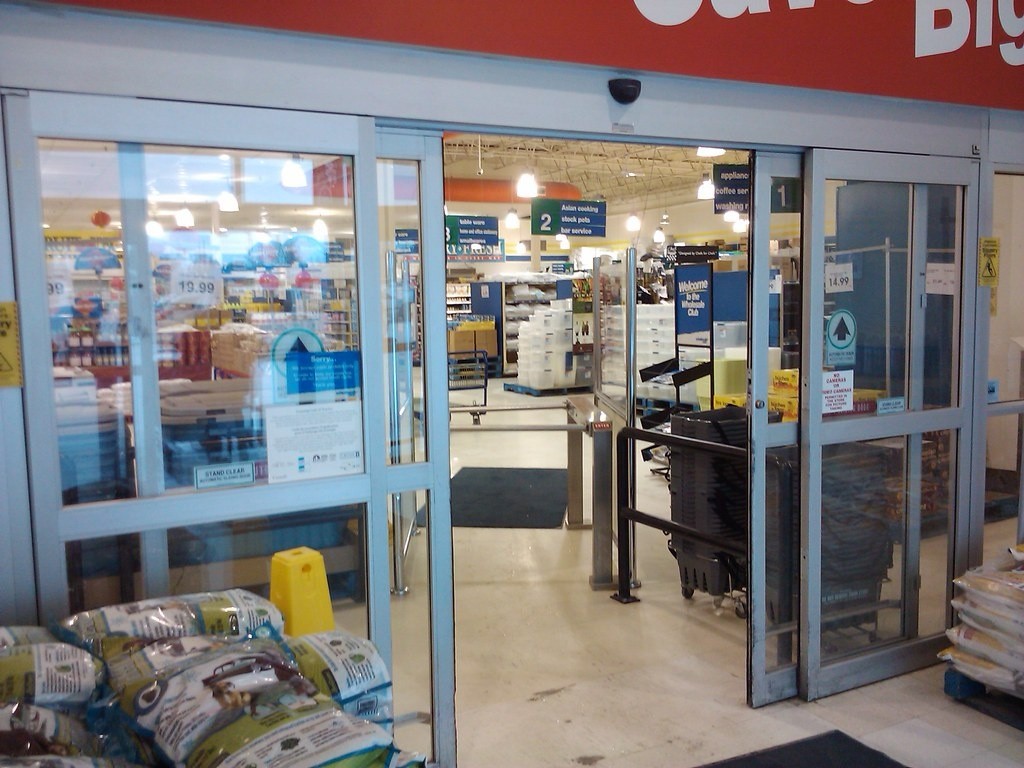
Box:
[53,348,129,367]
[68,323,127,348]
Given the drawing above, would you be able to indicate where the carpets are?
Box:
[417,466,568,530]
[693,729,910,768]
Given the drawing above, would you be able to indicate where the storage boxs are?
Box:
[710,260,733,272]
[476,329,498,357]
[770,257,793,280]
[448,330,476,358]
[770,239,790,255]
[601,304,952,642]
[518,300,594,389]
[211,333,345,375]
[738,259,747,271]
[710,239,724,246]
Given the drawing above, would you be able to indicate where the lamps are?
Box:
[506,208,520,228]
[660,192,671,225]
[733,219,746,233]
[696,146,726,156]
[556,234,570,250]
[626,211,641,231]
[724,211,740,222]
[696,179,715,199]
[654,227,665,243]
[516,241,527,254]
[140,151,330,243]
[516,168,538,198]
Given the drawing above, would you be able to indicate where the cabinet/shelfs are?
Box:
[412,283,472,367]
[557,278,651,393]
[323,299,357,350]
[470,280,556,374]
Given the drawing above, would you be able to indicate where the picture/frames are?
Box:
[481,285,489,298]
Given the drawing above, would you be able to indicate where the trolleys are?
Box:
[413,349,489,425]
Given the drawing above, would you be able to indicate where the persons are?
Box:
[153,266,180,321]
